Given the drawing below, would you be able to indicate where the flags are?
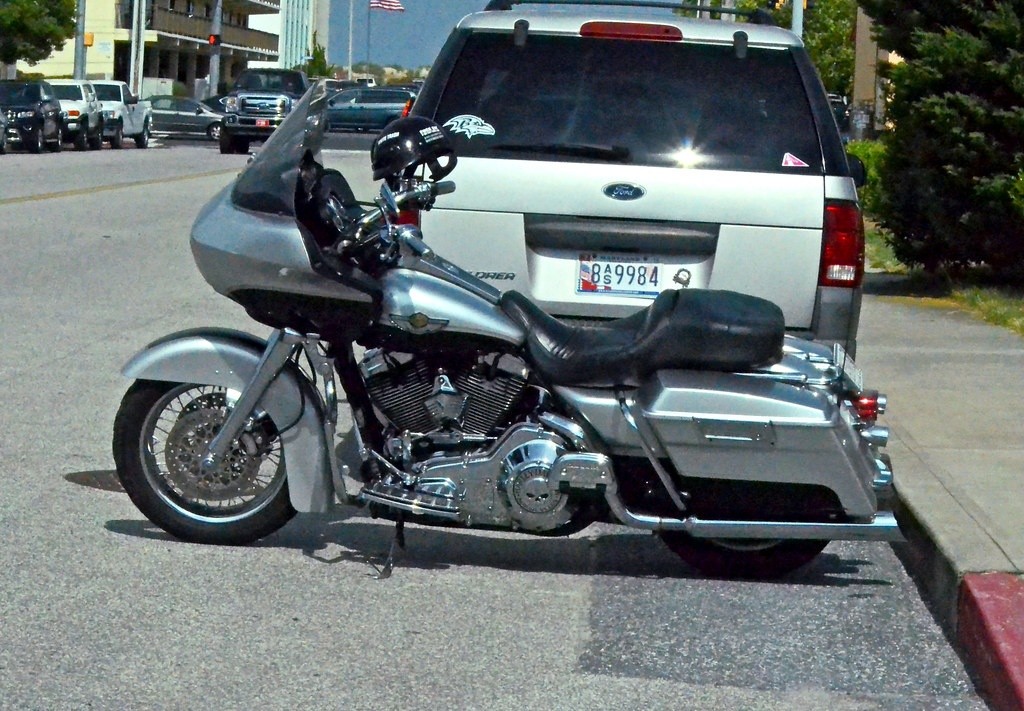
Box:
[370,0,404,12]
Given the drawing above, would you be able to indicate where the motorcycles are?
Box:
[110,77,904,578]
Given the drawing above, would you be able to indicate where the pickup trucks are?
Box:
[90,79,153,150]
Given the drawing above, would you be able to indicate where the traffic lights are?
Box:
[209,35,221,47]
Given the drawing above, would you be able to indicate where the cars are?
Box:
[320,86,419,133]
[0,79,65,151]
[145,96,224,140]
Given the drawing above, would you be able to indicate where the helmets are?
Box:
[370,116,458,191]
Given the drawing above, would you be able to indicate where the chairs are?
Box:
[245,75,263,89]
[480,71,554,127]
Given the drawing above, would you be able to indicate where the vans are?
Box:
[308,78,337,103]
[356,78,378,88]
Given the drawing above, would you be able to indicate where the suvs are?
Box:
[45,79,105,151]
[380,1,866,373]
[218,68,311,155]
